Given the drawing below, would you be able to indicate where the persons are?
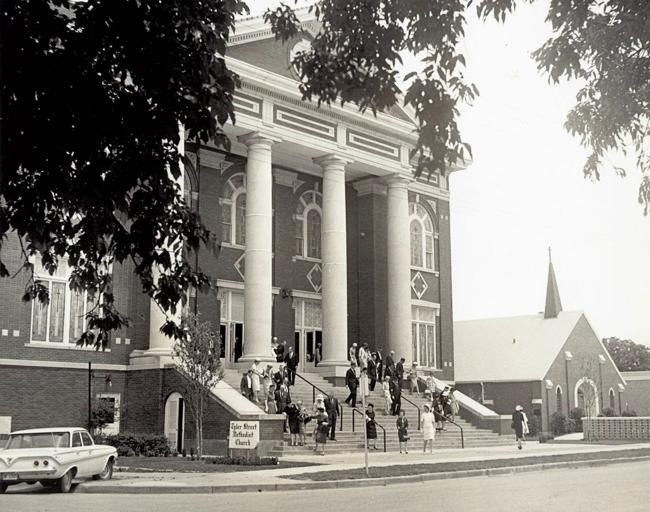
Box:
[520,407,529,440]
[240,336,459,454]
[513,405,524,449]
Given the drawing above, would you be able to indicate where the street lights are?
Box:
[87,359,113,442]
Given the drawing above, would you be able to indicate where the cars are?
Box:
[1,428,118,496]
